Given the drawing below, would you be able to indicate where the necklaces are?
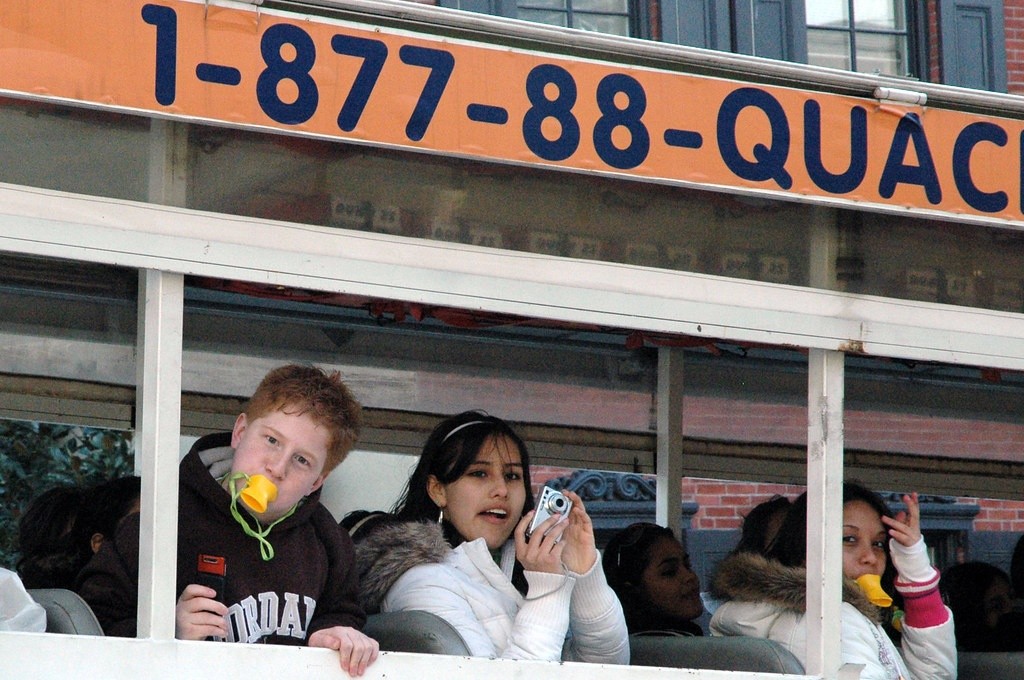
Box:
[865,618,899,677]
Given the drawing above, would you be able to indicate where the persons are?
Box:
[0,365,1024,680]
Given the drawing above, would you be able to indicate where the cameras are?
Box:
[525,486,573,546]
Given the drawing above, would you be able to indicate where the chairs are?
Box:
[27,588,1024,680]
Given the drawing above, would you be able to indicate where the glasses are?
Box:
[617,522,659,566]
[983,589,1016,616]
